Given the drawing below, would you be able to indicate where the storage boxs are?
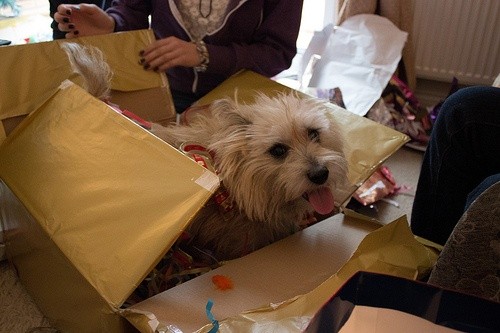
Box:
[0,29,444,333]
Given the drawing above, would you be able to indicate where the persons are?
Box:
[426,180,500,302]
[49,0,111,40]
[54,0,302,109]
[410,75,500,247]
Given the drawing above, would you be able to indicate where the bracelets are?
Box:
[191,40,209,72]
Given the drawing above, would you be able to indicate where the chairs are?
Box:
[411,87,500,247]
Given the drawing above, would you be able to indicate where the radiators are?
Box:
[398,0,500,87]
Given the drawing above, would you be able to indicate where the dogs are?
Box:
[57,38,352,295]
